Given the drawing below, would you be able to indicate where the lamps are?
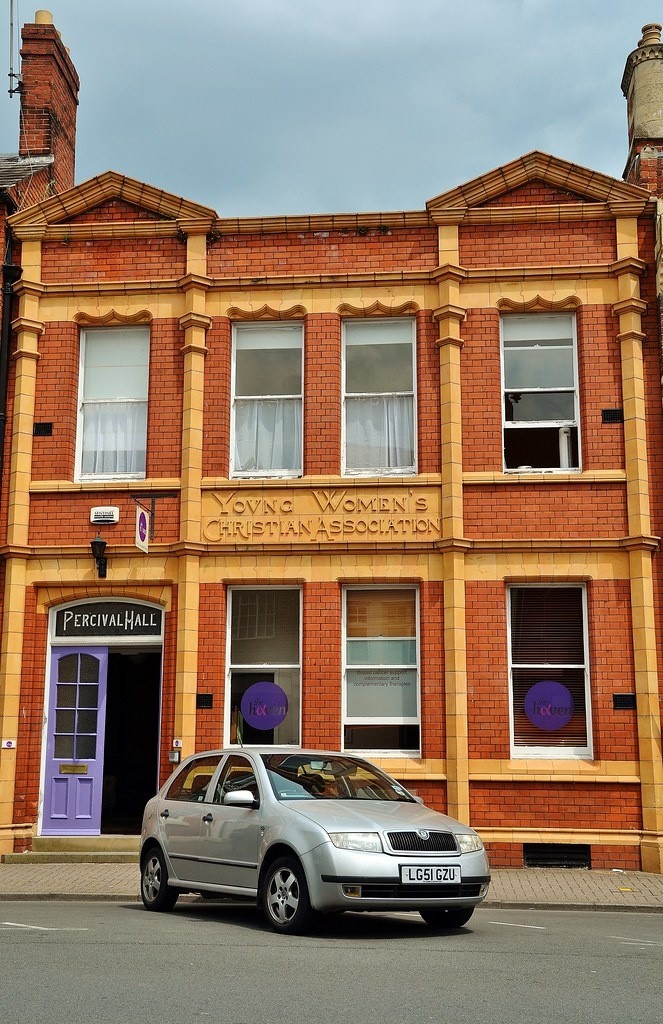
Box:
[90,531,109,579]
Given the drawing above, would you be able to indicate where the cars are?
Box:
[138,746,492,935]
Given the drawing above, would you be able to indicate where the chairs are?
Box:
[191,771,327,802]
